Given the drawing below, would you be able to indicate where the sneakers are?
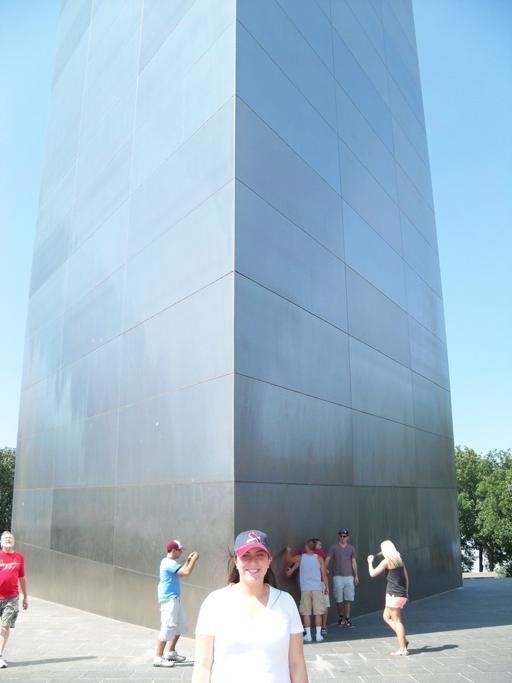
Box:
[152,658,174,668]
[303,618,351,640]
[166,651,185,661]
[0,656,7,667]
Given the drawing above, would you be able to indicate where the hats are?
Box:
[232,530,273,562]
[338,529,348,534]
[167,540,184,552]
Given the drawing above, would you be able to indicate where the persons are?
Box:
[325,528,360,629]
[0,530,31,669]
[152,539,201,667]
[193,529,308,682]
[367,539,410,657]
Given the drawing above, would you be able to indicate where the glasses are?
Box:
[339,536,347,538]
